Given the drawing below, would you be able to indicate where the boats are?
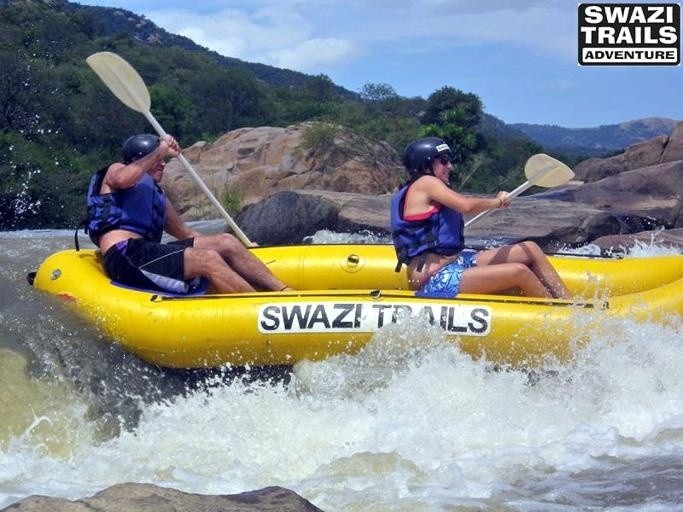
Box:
[24,241,683,373]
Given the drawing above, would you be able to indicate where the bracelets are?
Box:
[496,198,502,209]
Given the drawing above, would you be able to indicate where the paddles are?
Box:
[86,52,258,248]
[464,154,574,228]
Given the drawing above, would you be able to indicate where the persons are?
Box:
[389,136,579,301]
[83,131,297,294]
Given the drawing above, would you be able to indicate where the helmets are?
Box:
[400,137,454,177]
[120,134,161,161]
